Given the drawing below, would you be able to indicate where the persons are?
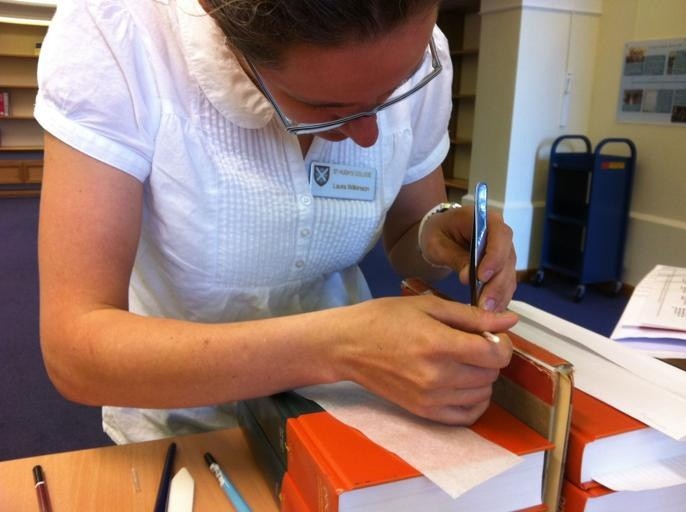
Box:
[33,1,518,447]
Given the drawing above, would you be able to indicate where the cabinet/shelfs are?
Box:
[1,21,49,197]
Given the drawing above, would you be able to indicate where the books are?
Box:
[558,384,686,512]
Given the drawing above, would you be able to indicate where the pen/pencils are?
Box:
[204,449,259,512]
[34,466,52,511]
[153,442,177,512]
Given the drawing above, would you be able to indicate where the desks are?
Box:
[0,428,282,512]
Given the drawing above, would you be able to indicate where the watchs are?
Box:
[419,200,464,271]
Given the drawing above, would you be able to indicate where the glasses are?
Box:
[242,35,443,134]
